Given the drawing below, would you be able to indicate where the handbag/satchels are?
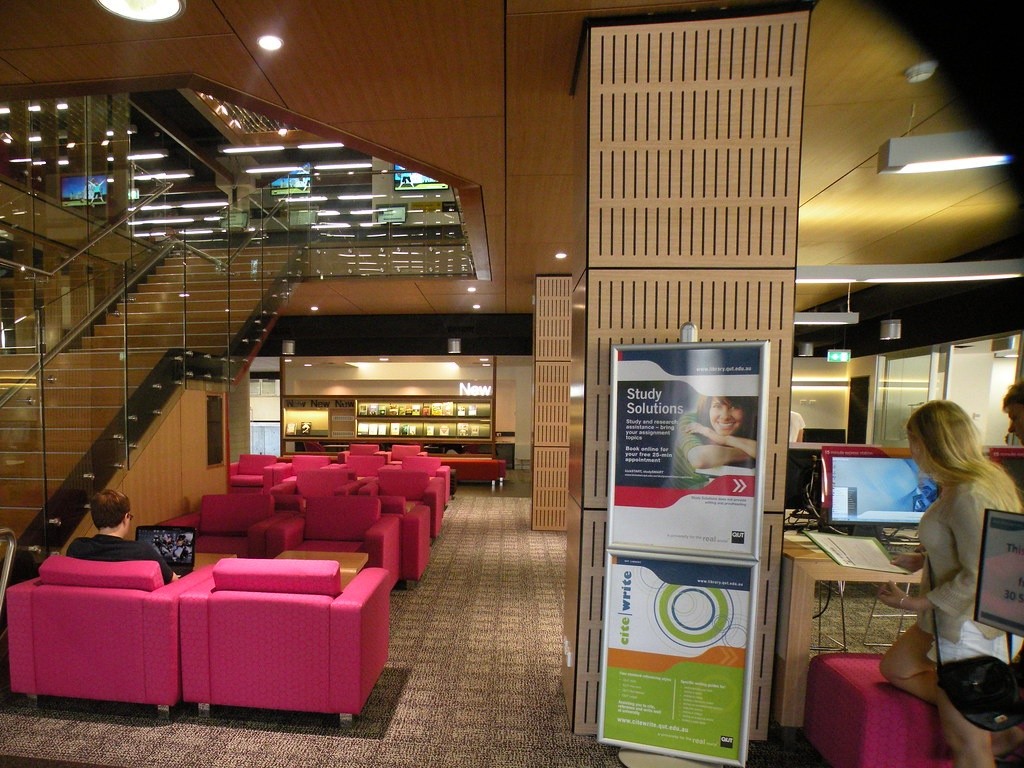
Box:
[937,655,1024,731]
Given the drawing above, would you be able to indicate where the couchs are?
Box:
[5,445,456,723]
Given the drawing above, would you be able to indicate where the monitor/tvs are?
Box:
[61,174,108,206]
[393,164,450,190]
[128,188,139,199]
[789,445,941,539]
[270,161,312,196]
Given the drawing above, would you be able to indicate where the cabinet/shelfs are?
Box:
[281,397,493,457]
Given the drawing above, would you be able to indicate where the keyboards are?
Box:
[881,544,923,555]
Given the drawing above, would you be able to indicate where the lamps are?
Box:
[875,59,1015,174]
[448,338,461,354]
[880,309,901,340]
[282,340,295,355]
[797,342,814,357]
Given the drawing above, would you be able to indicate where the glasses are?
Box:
[127,513,133,520]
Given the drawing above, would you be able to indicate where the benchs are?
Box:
[441,460,505,485]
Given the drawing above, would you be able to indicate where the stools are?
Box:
[802,652,1024,768]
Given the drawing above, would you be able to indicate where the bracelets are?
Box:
[900,594,909,609]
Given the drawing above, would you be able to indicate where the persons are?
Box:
[66,490,178,585]
[876,400,1024,768]
[1003,381,1024,445]
[789,411,806,443]
[664,395,758,488]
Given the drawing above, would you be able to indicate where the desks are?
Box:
[695,466,754,482]
[194,553,236,569]
[274,551,369,589]
[772,530,923,728]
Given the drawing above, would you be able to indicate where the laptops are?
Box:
[135,525,197,580]
